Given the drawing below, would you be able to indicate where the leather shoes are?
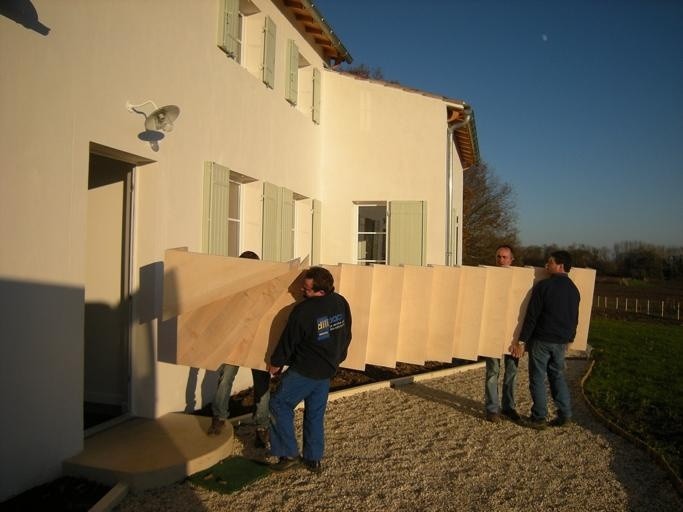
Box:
[207,418,225,436]
[255,431,268,449]
[271,455,321,472]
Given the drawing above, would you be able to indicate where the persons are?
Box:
[512,250,581,430]
[484,244,520,421]
[269,268,353,474]
[206,250,274,450]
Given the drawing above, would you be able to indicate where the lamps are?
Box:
[125,96,182,133]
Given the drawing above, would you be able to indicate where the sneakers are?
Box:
[518,417,571,430]
[486,409,521,423]
